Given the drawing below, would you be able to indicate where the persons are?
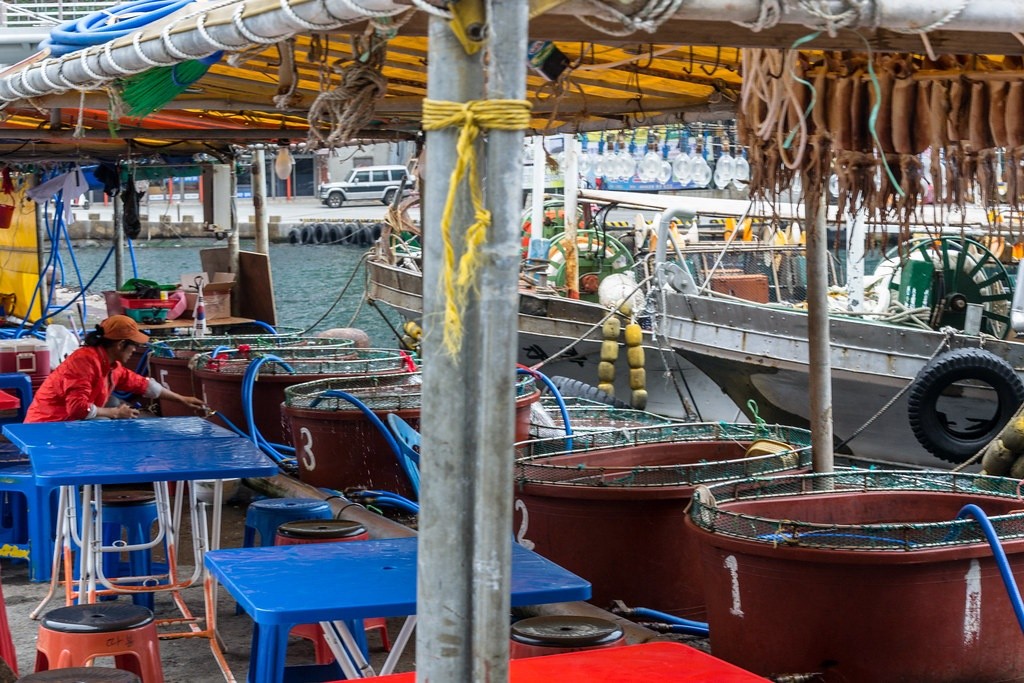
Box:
[23,314,208,423]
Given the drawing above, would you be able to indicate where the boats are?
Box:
[362,145,1024,478]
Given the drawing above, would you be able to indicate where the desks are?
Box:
[27,437,279,668]
[138,317,256,335]
[204,536,592,683]
[0,415,240,621]
[329,641,773,683]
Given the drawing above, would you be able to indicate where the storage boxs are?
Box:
[101,291,137,315]
[0,338,50,399]
[710,275,769,304]
[125,308,171,323]
[178,272,237,320]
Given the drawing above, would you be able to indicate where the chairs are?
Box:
[387,413,515,543]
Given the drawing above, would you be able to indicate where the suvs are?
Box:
[316,165,416,208]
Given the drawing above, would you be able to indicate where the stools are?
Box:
[275,519,391,665]
[0,372,33,434]
[73,490,170,613]
[34,602,165,683]
[0,464,77,584]
[15,667,142,683]
[510,616,626,659]
[236,497,334,617]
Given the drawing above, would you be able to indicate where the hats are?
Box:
[98,314,150,345]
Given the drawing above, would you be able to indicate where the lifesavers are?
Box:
[908,345,1024,464]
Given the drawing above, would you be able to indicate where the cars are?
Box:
[55,193,91,210]
